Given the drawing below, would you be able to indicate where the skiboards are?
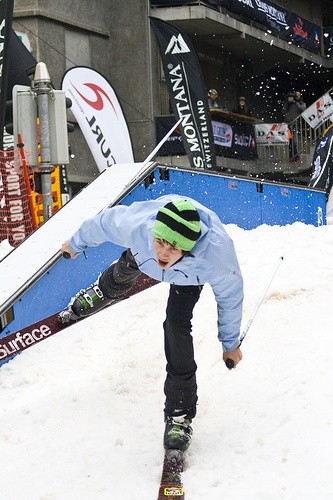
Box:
[0,272,185,500]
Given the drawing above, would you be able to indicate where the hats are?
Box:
[150,199,201,251]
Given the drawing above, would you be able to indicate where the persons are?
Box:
[60,193,243,451]
[232,95,249,116]
[207,88,218,108]
[281,91,306,161]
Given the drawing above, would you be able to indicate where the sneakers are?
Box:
[71,286,113,316]
[163,410,190,450]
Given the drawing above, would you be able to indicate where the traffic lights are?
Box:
[47,89,73,165]
[5,92,38,166]
